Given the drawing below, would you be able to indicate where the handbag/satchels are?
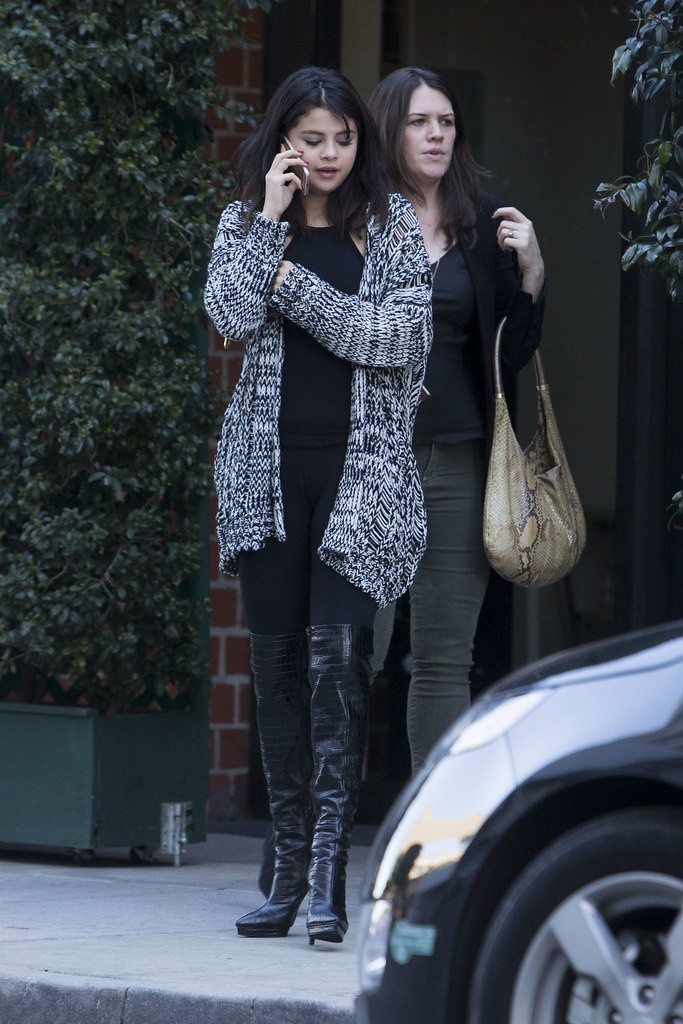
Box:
[483,316,586,588]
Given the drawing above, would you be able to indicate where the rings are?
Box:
[508,230,513,238]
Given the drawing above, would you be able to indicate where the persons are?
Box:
[204,69,431,941]
[259,68,545,903]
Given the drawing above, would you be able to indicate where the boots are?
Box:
[235,630,311,938]
[306,624,375,948]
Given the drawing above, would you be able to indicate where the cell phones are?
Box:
[283,135,310,197]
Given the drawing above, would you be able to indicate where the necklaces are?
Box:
[431,239,441,281]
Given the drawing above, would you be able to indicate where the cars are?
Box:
[350,615,683,1024]
